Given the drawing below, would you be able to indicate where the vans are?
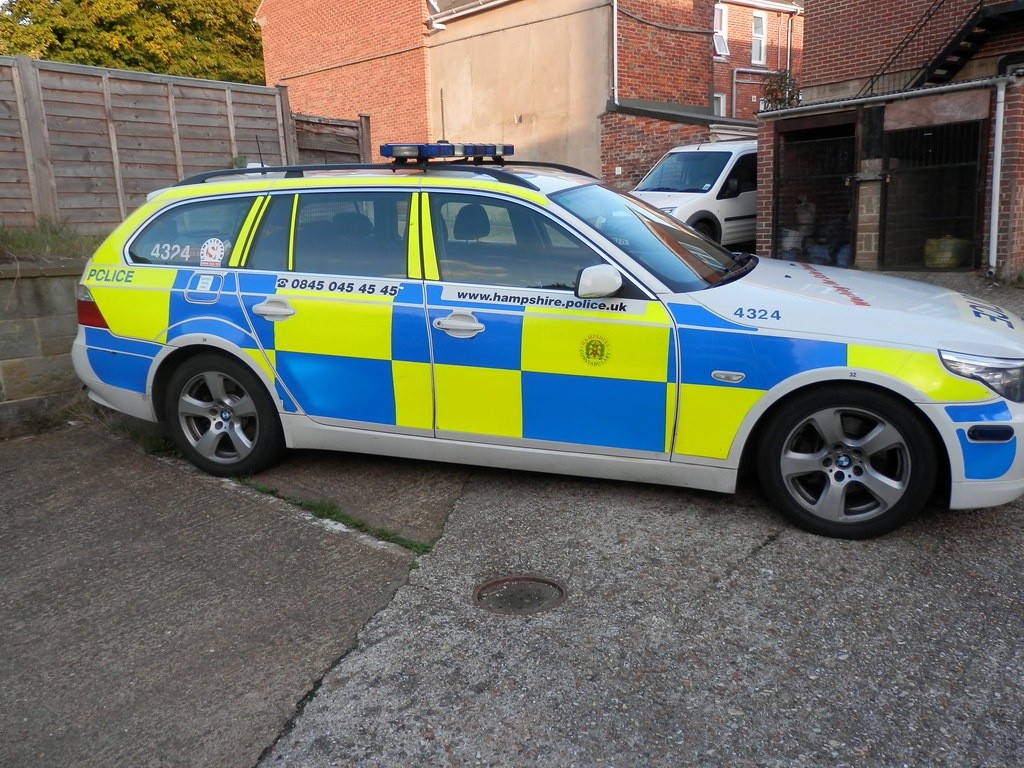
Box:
[622,141,758,247]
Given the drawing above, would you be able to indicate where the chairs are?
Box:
[407,210,458,281]
[452,205,518,286]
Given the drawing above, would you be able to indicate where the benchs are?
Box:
[265,212,407,278]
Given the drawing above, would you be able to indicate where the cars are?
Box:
[69,136,1024,540]
